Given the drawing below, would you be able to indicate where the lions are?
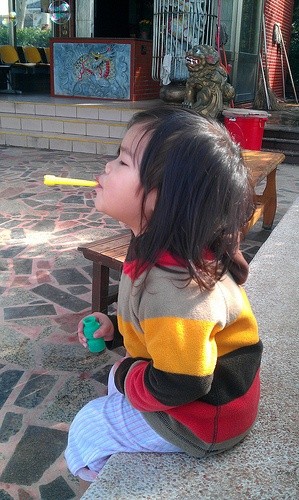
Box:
[182,43,234,120]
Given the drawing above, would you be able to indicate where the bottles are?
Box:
[83,315,106,353]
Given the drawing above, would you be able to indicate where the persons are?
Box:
[64,106,263,481]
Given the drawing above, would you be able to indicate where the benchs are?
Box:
[76,232,132,313]
[0,45,51,94]
[236,147,285,240]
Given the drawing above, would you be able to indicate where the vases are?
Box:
[141,33,150,39]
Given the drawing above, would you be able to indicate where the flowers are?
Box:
[139,20,152,33]
